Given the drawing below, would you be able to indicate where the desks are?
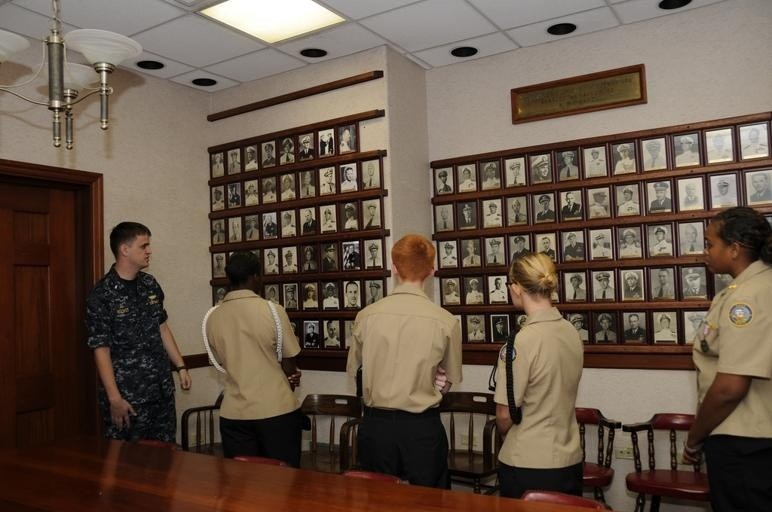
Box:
[0,438,610,512]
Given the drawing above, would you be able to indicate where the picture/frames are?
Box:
[510,63,648,125]
[209,121,387,351]
[434,120,771,347]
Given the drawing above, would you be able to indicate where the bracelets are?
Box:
[176,365,189,372]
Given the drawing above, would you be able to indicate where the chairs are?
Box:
[180,391,228,457]
[623,413,707,512]
[435,392,501,494]
[298,393,365,470]
[580,409,622,506]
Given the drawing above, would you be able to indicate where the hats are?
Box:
[437,127,769,326]
[214,131,381,330]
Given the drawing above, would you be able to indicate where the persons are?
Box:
[348,233,462,491]
[432,122,771,346]
[205,125,383,349]
[682,207,771,510]
[488,252,586,502]
[200,249,310,469]
[83,220,192,442]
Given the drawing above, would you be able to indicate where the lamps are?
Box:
[0,0,143,149]
